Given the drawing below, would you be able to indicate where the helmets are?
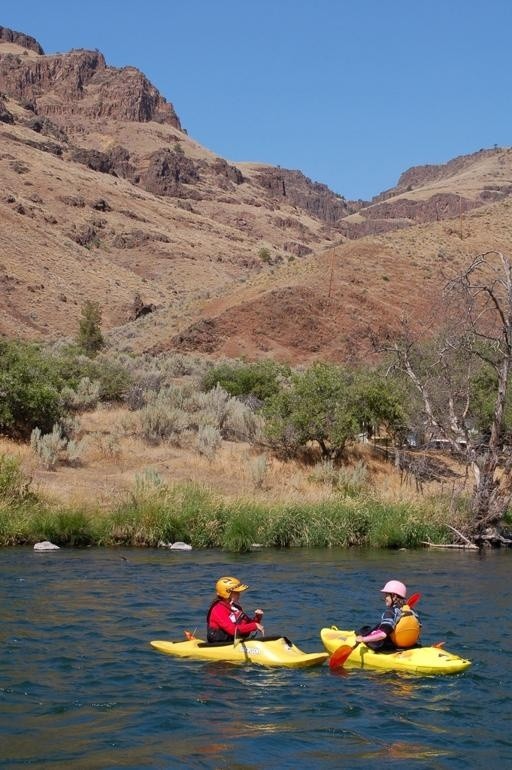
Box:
[379,580,407,599]
[215,576,249,600]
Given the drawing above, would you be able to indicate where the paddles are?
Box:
[329,592,421,670]
[234,627,258,649]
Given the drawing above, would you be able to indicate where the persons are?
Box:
[207,576,265,643]
[355,580,422,653]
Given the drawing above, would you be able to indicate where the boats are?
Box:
[149,629,330,670]
[320,625,473,680]
